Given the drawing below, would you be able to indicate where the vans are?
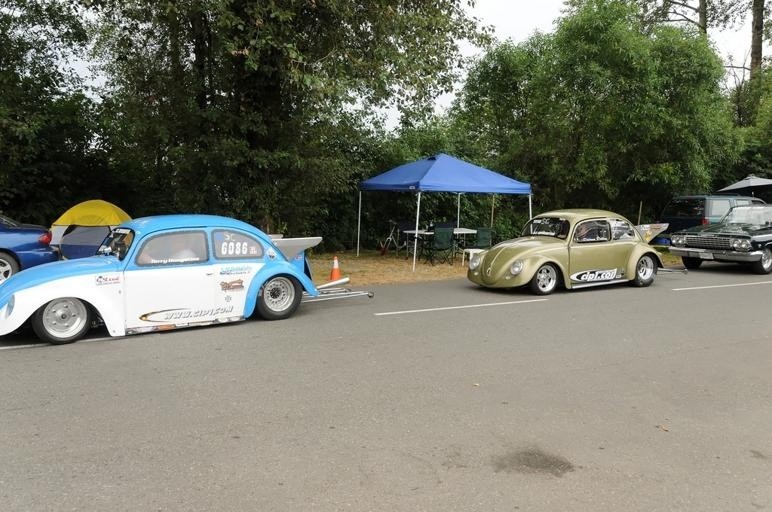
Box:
[664,195,766,235]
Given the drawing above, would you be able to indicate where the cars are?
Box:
[467,208,669,295]
[669,204,772,274]
[0,212,323,344]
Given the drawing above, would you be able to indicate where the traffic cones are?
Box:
[330,256,342,280]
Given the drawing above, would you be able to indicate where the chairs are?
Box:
[375,206,492,266]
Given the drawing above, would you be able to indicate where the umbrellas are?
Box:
[717,173,772,199]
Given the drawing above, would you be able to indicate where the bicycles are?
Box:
[381,220,424,257]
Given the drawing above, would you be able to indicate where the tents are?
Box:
[47,200,133,260]
